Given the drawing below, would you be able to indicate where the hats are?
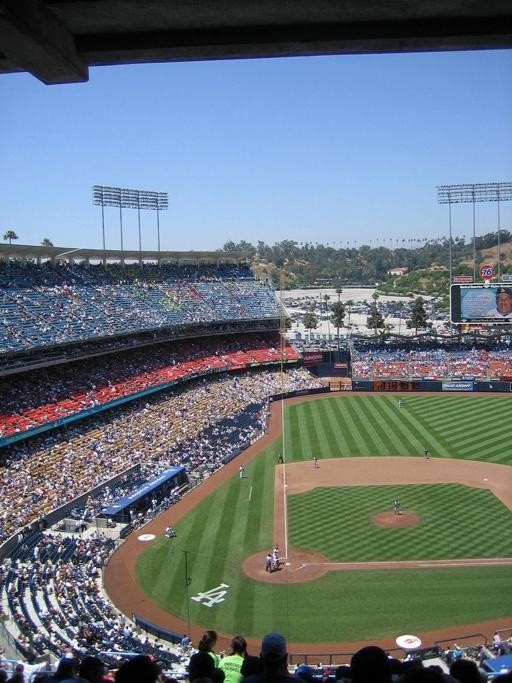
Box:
[295,664,311,676]
[261,633,286,661]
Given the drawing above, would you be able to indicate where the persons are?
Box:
[277,454,284,463]
[399,399,403,407]
[240,465,245,479]
[424,448,432,457]
[165,526,176,538]
[487,287,510,318]
[2,531,199,681]
[393,500,400,515]
[313,456,318,468]
[265,546,283,574]
[2,265,328,544]
[188,631,510,681]
[329,340,510,381]
